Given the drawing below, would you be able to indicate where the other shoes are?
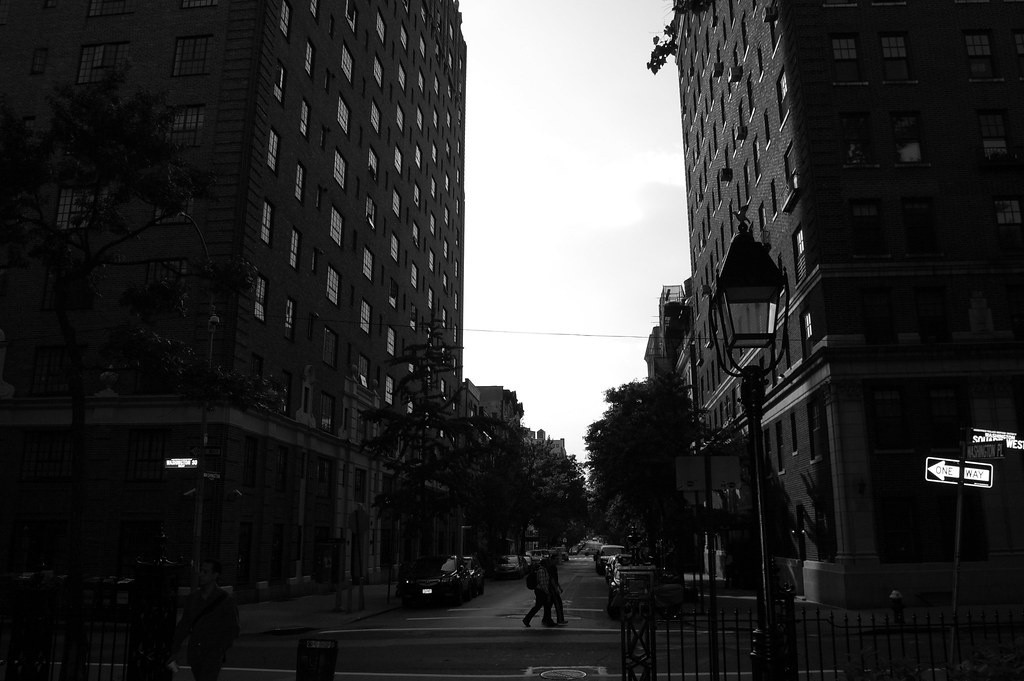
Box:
[557,621,568,625]
[523,618,530,627]
[542,619,556,627]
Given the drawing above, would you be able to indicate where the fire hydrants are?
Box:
[889,589,906,624]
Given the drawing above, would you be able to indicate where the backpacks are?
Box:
[526,567,546,589]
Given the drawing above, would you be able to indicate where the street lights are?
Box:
[700,202,802,681]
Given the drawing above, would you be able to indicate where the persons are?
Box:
[522,554,568,628]
[155,556,241,681]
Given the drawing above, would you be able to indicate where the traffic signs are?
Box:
[963,427,1024,461]
[924,456,994,489]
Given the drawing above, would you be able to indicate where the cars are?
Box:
[398,553,485,611]
[490,546,570,581]
[585,542,661,621]
